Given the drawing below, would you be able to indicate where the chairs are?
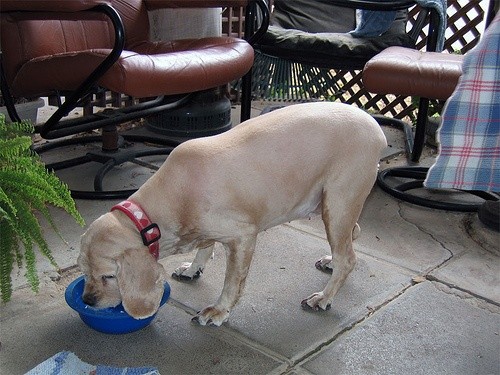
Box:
[240,0,446,161]
[0,0,270,200]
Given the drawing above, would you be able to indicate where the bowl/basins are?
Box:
[64,275,171,335]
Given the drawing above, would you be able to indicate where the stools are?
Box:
[361,45,500,212]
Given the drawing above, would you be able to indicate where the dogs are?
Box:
[76,101,389,328]
